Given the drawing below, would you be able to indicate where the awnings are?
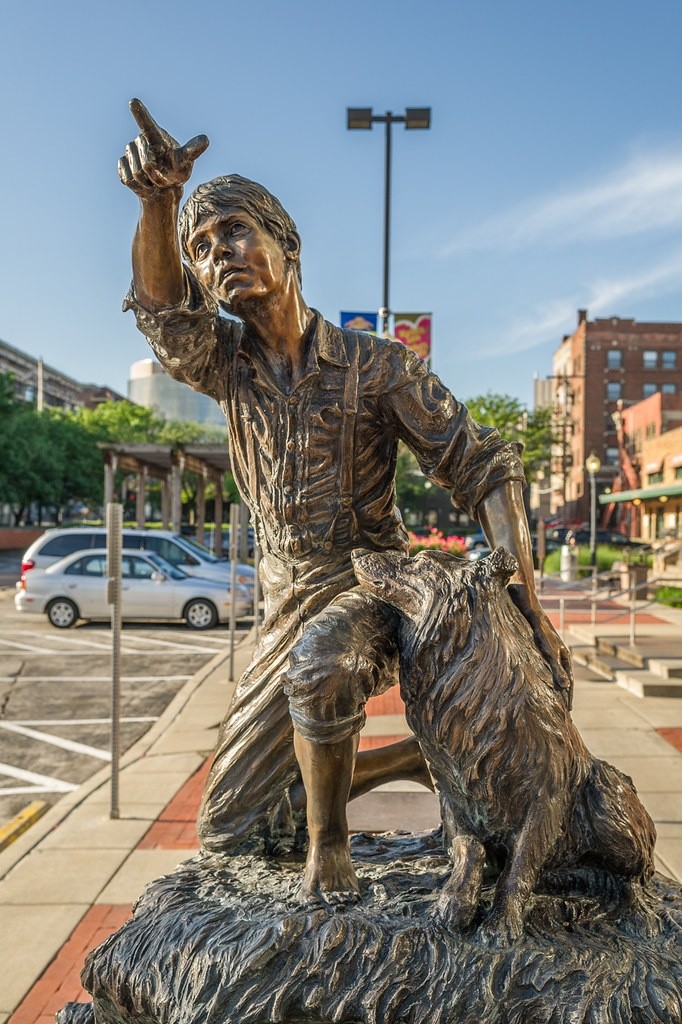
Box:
[598,483,681,504]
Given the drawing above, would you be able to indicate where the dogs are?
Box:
[351,547,657,944]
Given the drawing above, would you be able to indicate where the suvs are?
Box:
[566,530,648,550]
[530,536,560,569]
[21,529,262,600]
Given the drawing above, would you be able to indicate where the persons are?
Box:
[118,98,574,905]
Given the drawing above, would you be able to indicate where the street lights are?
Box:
[346,106,430,343]
[584,450,600,567]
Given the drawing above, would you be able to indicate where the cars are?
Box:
[463,533,561,568]
[192,532,254,562]
[14,548,251,629]
